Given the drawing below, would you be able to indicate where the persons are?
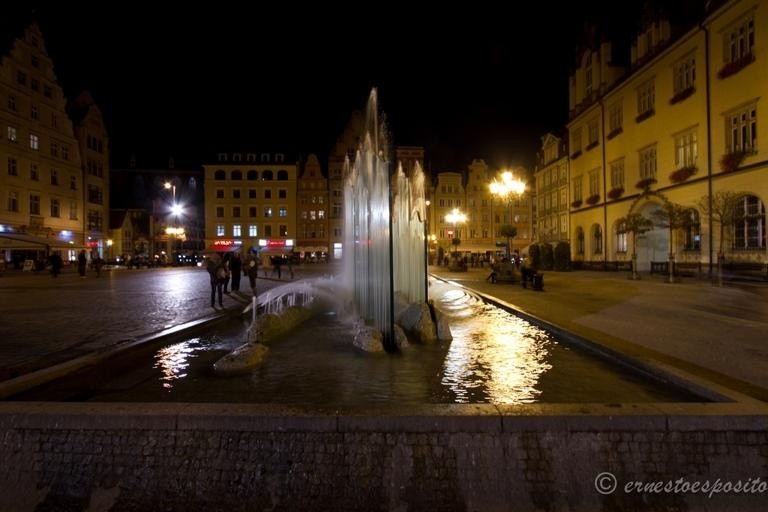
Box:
[95,256,101,278]
[207,253,224,306]
[485,260,499,284]
[57,255,61,274]
[231,252,241,292]
[77,250,88,279]
[242,253,295,280]
[51,251,58,278]
[520,254,529,287]
[244,249,258,298]
[222,253,232,294]
[428,250,495,270]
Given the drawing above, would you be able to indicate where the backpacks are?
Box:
[212,258,226,280]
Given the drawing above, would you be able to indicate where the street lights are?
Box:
[489,171,526,284]
[443,208,468,272]
